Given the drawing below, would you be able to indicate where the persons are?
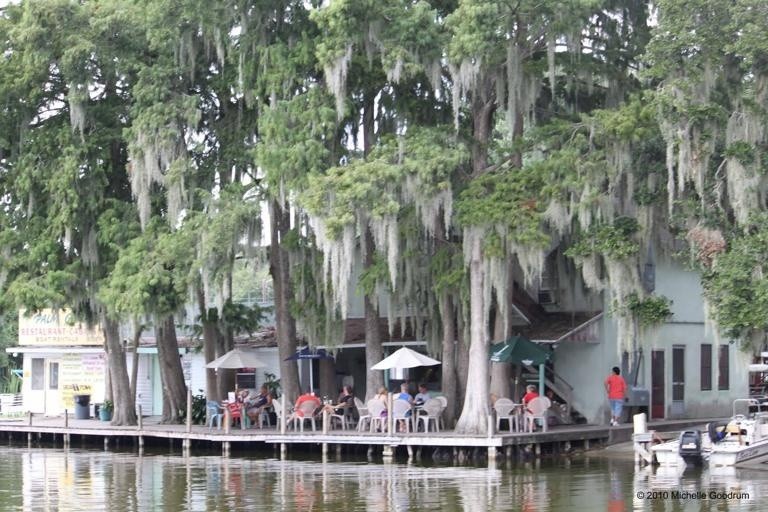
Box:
[413,383,433,432]
[246,385,271,425]
[392,383,414,432]
[293,385,320,417]
[317,384,353,427]
[490,393,497,406]
[604,366,628,426]
[373,387,390,432]
[522,385,541,432]
[230,389,249,429]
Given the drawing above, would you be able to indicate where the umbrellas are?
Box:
[370,345,442,392]
[283,345,335,394]
[486,333,546,402]
[203,347,268,393]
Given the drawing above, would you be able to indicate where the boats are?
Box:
[651,350,767,476]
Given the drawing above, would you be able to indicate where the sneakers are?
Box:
[608,418,620,428]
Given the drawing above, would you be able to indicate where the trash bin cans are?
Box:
[74,395,90,419]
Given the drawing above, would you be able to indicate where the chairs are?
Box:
[205,387,448,435]
[493,395,552,434]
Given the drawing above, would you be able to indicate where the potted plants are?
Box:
[96,397,114,421]
